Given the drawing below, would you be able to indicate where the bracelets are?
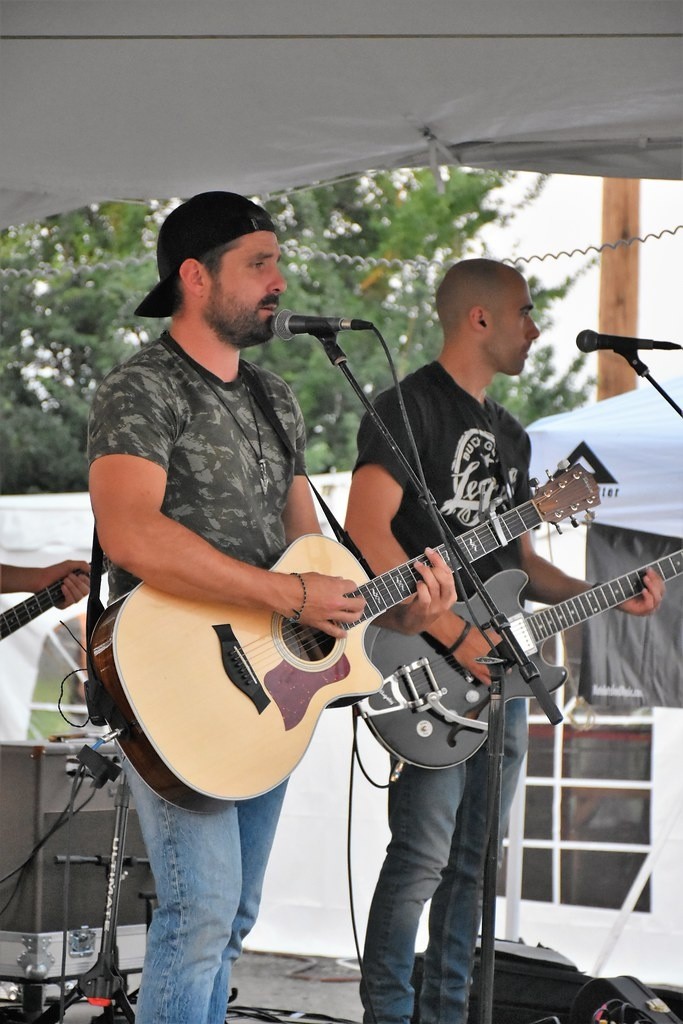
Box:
[287,571,308,624]
[449,620,472,654]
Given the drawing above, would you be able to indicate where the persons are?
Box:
[341,257,663,1024]
[0,561,90,609]
[89,189,455,1024]
[345,259,667,1024]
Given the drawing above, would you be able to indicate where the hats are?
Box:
[134,190,279,319]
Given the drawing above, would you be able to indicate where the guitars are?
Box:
[79,455,609,820]
[350,544,683,771]
[0,553,119,645]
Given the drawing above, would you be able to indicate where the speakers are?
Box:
[569,976,683,1024]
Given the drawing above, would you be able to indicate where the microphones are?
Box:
[575,329,682,353]
[269,309,374,341]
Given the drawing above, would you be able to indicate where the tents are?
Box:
[0,378,683,991]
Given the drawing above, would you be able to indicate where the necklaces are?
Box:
[196,370,270,496]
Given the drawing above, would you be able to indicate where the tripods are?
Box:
[32,775,134,1024]
